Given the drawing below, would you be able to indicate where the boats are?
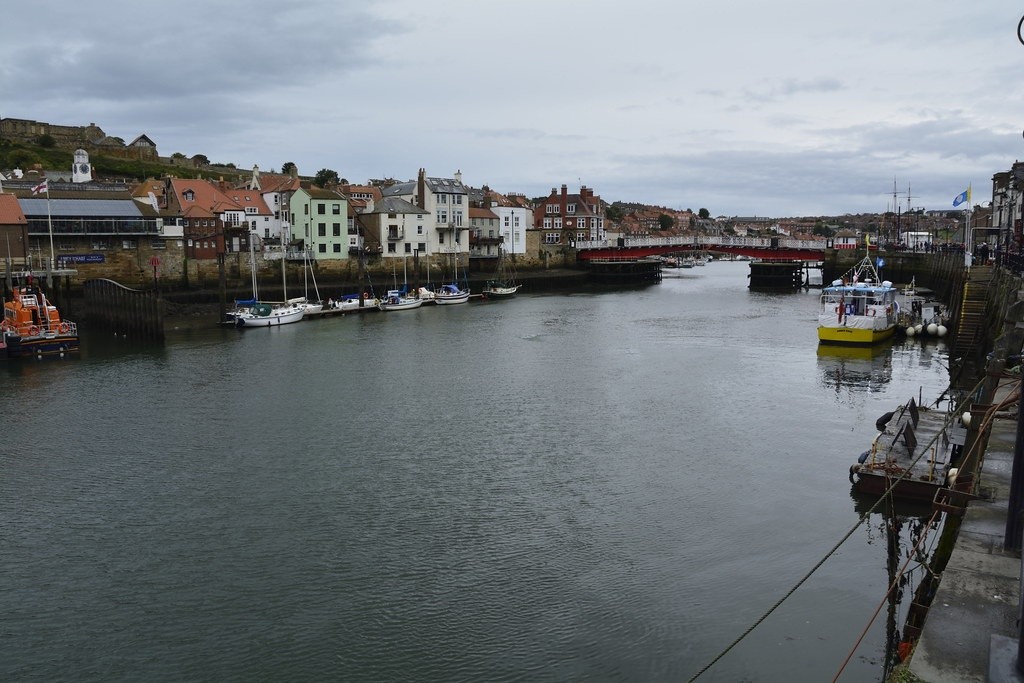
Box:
[856,402,959,503]
[817,233,900,346]
[0,276,81,357]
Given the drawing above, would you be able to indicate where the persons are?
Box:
[980,242,989,265]
[912,299,922,318]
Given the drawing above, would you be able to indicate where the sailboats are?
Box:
[377,249,436,311]
[433,223,471,305]
[482,240,523,300]
[329,224,380,313]
[224,199,322,328]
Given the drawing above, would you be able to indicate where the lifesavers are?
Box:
[58,322,69,333]
[28,325,39,336]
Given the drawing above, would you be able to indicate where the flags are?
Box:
[876,257,885,267]
[31,180,47,195]
[952,190,967,207]
